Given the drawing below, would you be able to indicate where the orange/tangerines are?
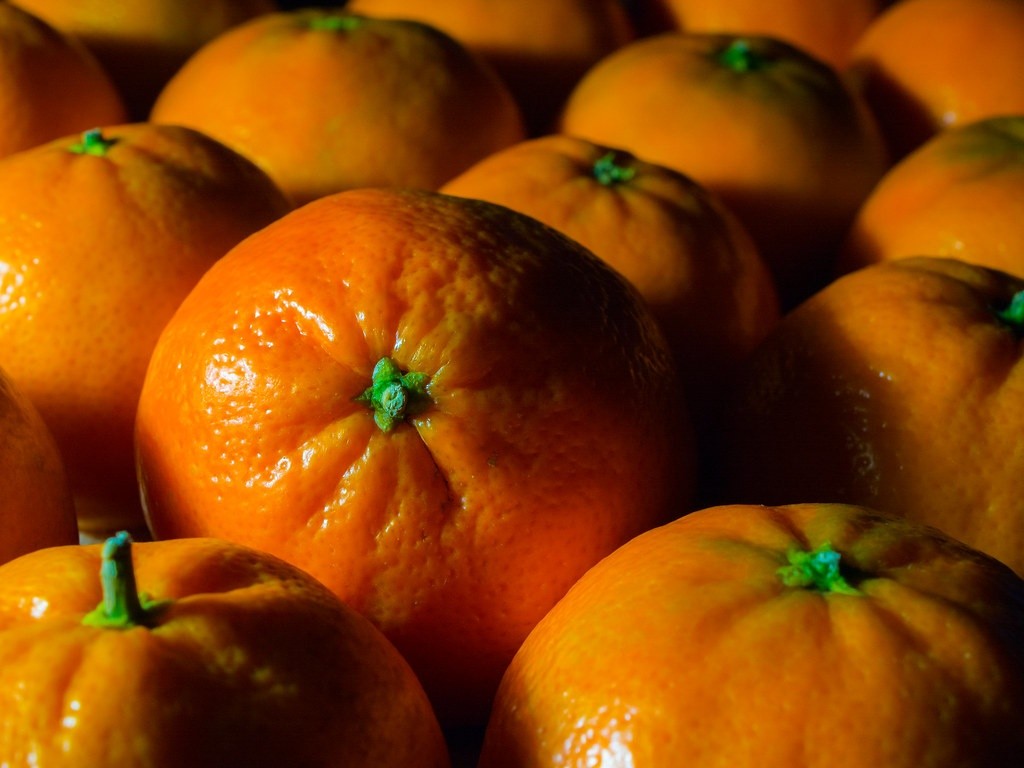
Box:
[2,0,1023,767]
